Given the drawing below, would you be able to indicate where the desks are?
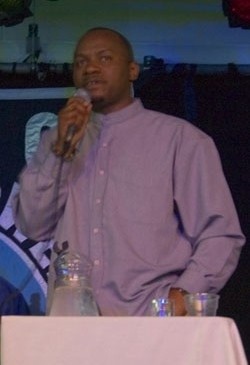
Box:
[0,314,250,365]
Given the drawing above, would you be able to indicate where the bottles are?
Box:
[47,250,100,316]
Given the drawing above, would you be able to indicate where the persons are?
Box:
[12,28,247,316]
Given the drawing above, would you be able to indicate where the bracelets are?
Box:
[178,288,187,296]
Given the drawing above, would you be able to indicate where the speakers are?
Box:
[197,74,250,363]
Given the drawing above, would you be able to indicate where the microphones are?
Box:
[60,89,91,156]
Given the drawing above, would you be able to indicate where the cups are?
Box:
[184,292,221,316]
